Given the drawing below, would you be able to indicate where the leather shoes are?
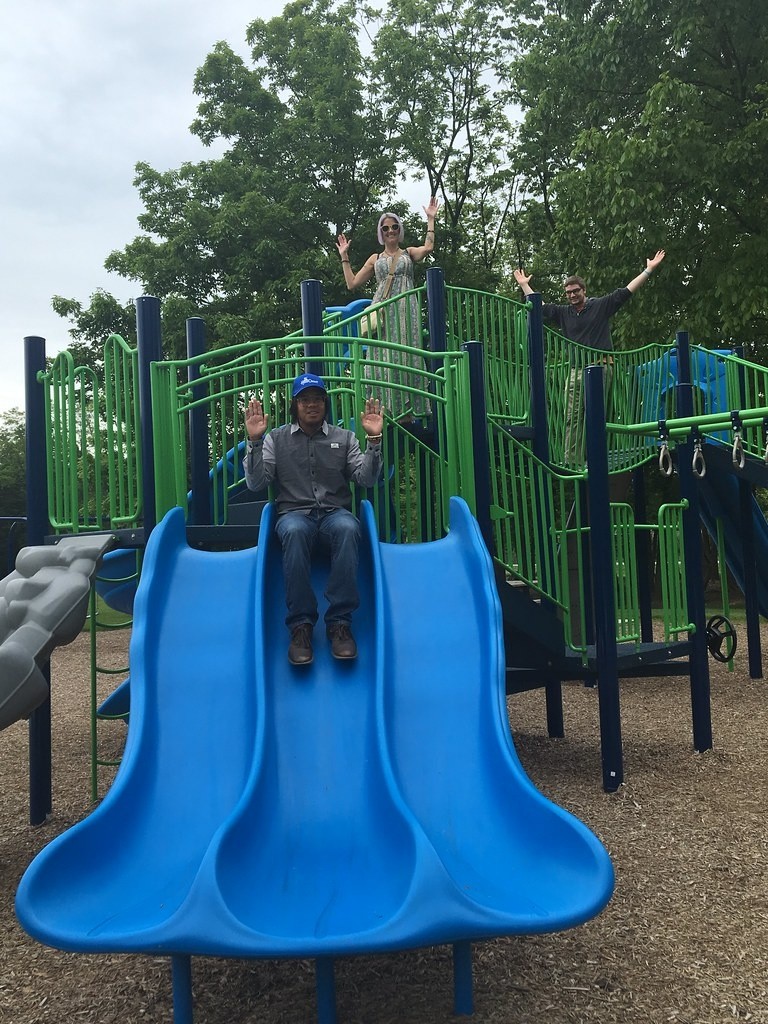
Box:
[288,622,314,665]
[326,623,358,660]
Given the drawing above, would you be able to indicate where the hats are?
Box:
[293,374,326,395]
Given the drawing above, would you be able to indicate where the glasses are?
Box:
[381,223,402,232]
[564,287,584,295]
[299,396,328,405]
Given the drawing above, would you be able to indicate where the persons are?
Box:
[242,374,385,665]
[335,196,441,423]
[513,250,666,470]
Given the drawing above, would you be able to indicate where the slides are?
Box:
[14,495,618,955]
[91,439,268,726]
[688,469,768,622]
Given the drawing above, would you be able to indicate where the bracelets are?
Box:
[427,230,435,233]
[341,260,350,263]
[366,433,382,441]
[644,270,650,276]
